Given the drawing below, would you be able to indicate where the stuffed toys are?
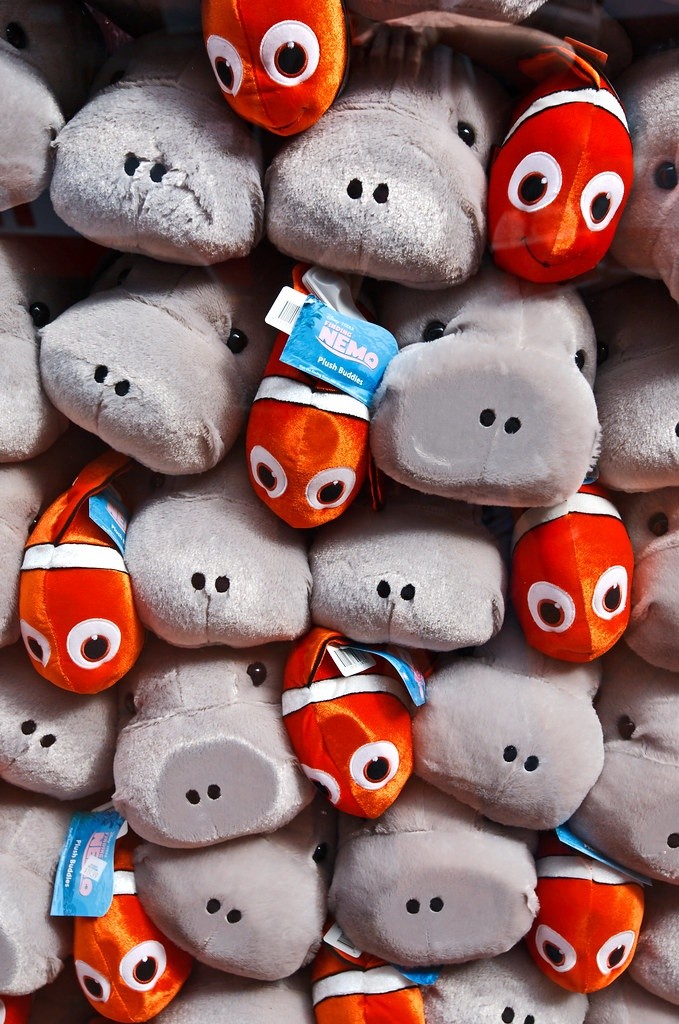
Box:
[0,0,679,1024]
